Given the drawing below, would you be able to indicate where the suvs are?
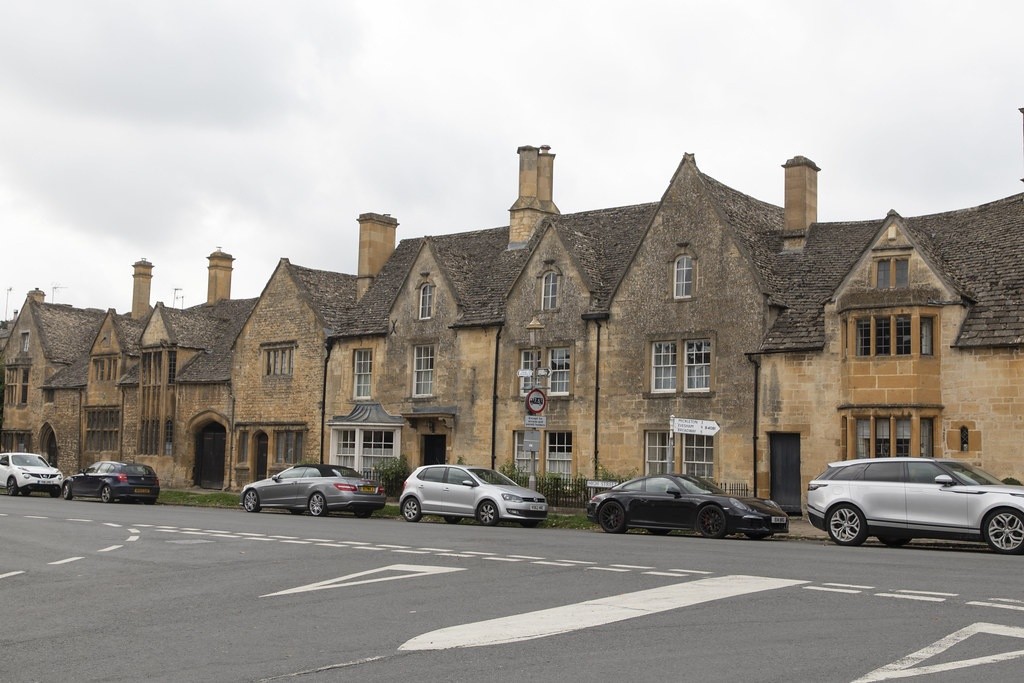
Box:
[805,456,1024,555]
[0,451,64,498]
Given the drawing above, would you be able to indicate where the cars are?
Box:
[586,471,792,540]
[239,463,388,519]
[398,463,550,528]
[61,460,160,505]
